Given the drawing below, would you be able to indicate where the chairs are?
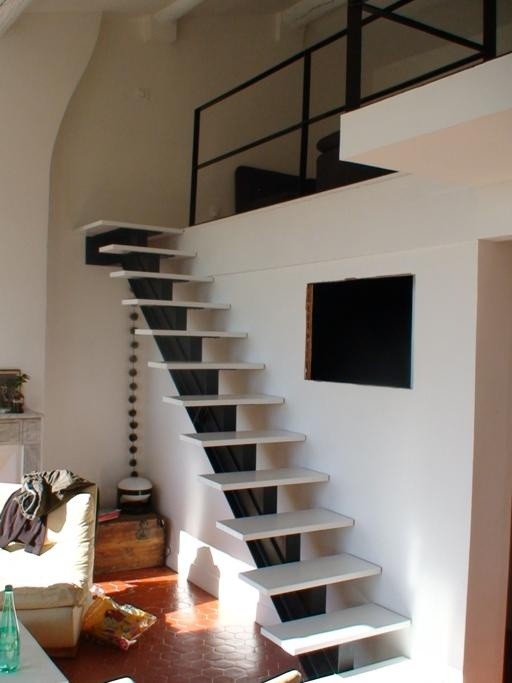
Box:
[0,481,98,659]
[233,129,398,213]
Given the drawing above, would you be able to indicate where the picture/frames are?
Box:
[0,369,22,409]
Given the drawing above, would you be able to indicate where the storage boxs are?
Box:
[93,509,166,574]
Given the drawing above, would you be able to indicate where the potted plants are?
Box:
[5,372,32,413]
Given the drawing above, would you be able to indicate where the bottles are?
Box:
[0,584,21,674]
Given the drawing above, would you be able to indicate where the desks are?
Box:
[0,612,72,683]
